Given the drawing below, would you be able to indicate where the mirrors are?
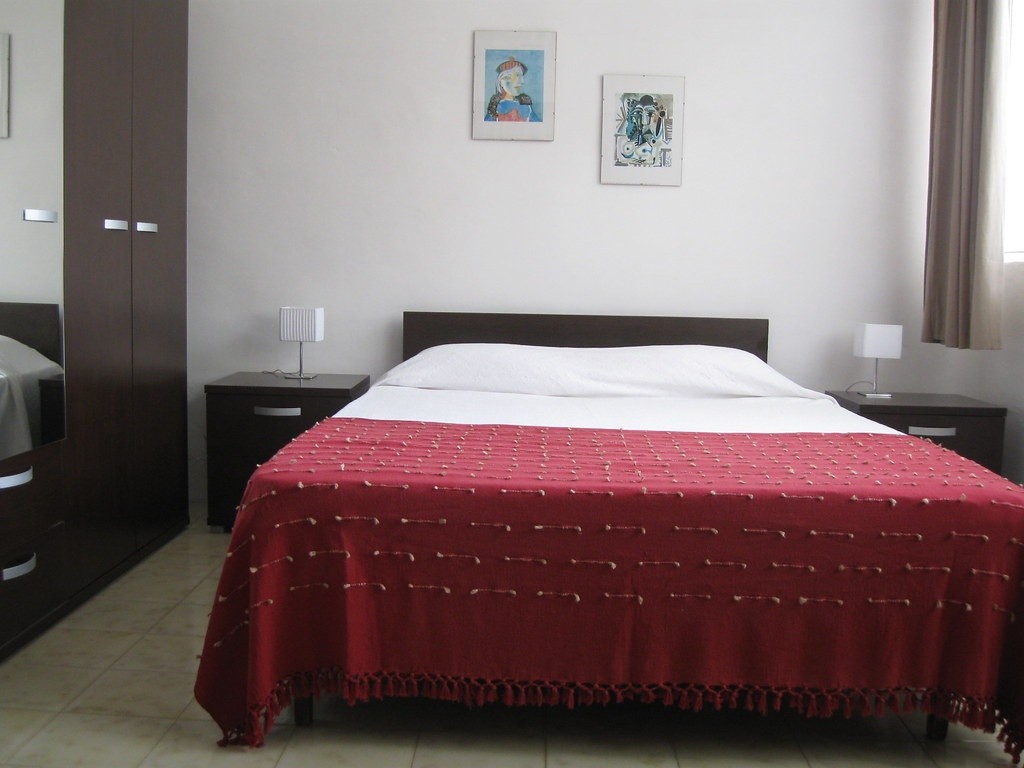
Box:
[0,0,67,462]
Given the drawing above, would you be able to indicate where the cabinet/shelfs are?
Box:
[0,0,189,666]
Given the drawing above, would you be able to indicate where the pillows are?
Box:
[375,344,826,397]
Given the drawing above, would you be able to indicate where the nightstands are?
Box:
[825,391,1008,477]
[204,372,371,534]
[38,374,65,446]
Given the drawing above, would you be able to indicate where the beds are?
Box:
[0,301,64,463]
[192,311,1024,768]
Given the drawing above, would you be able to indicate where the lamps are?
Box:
[852,322,902,396]
[280,306,325,379]
